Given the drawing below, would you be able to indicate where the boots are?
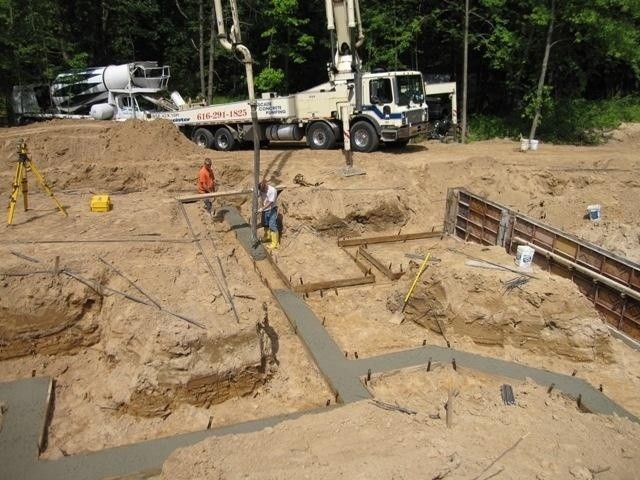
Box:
[261,228,271,242]
[266,232,279,248]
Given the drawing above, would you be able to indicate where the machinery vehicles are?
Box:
[7,60,172,127]
[137,1,459,249]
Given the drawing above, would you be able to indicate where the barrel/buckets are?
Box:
[520,139,530,150]
[515,245,536,268]
[530,139,539,150]
[587,204,602,221]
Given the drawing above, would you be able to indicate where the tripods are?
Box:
[7,154,68,224]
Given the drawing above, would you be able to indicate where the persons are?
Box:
[198,158,216,211]
[257,183,279,250]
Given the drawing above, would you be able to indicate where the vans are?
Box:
[422,73,481,125]
